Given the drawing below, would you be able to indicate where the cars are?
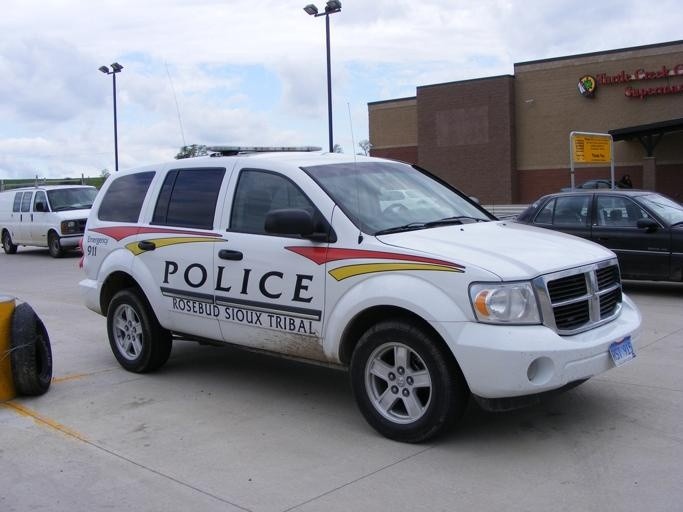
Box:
[558,177,639,192]
[497,189,682,286]
[380,187,443,222]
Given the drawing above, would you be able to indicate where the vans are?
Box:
[0,185,101,258]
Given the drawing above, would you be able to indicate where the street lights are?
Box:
[301,1,343,155]
[97,61,122,173]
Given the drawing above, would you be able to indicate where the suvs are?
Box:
[78,153,642,443]
